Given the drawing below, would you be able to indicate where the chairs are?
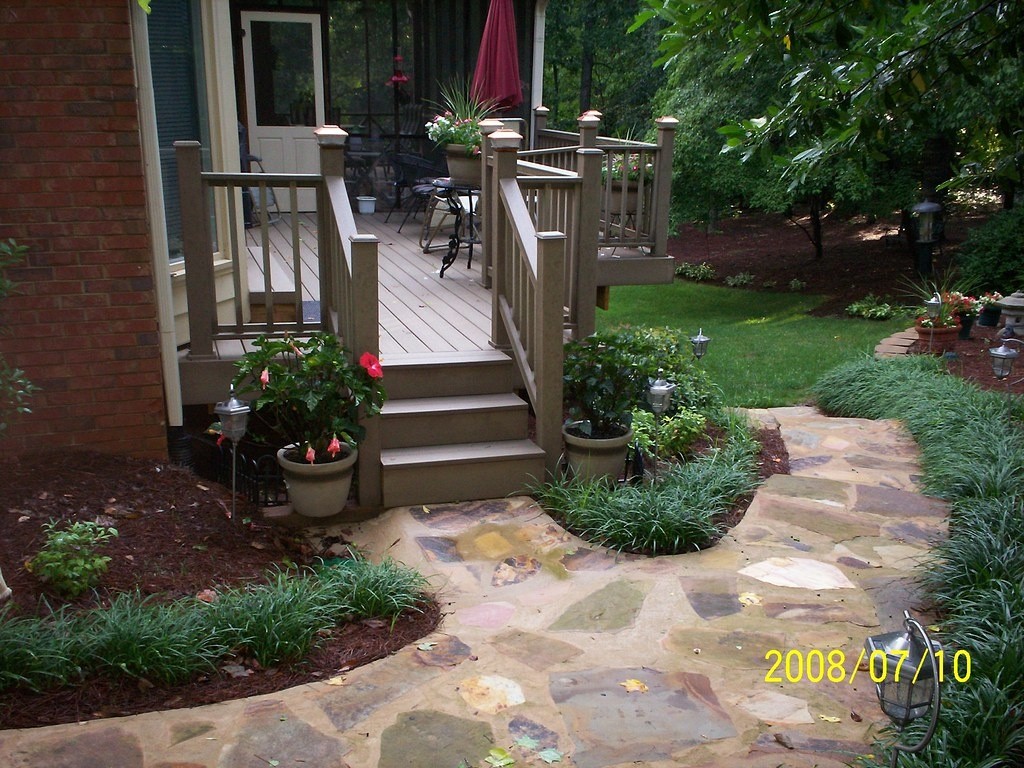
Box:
[239,122,281,227]
[383,152,481,255]
[333,103,430,197]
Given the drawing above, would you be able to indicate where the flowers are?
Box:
[204,330,387,465]
[975,291,1003,316]
[916,303,960,328]
[602,123,654,187]
[942,291,980,323]
[421,71,510,160]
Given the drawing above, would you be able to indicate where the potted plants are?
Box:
[561,331,665,489]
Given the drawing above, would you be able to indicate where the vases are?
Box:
[357,197,377,216]
[954,312,974,340]
[611,180,638,212]
[277,440,358,518]
[915,322,962,357]
[445,142,481,184]
[978,305,1001,327]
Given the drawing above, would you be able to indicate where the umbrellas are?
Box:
[471,0,525,115]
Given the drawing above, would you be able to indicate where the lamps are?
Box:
[213,384,251,446]
[862,609,953,733]
[689,328,711,362]
[646,368,676,413]
[988,340,1017,380]
[924,291,941,319]
[914,199,940,244]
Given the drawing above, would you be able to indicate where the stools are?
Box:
[601,210,644,238]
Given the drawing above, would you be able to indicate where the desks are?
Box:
[432,178,481,278]
